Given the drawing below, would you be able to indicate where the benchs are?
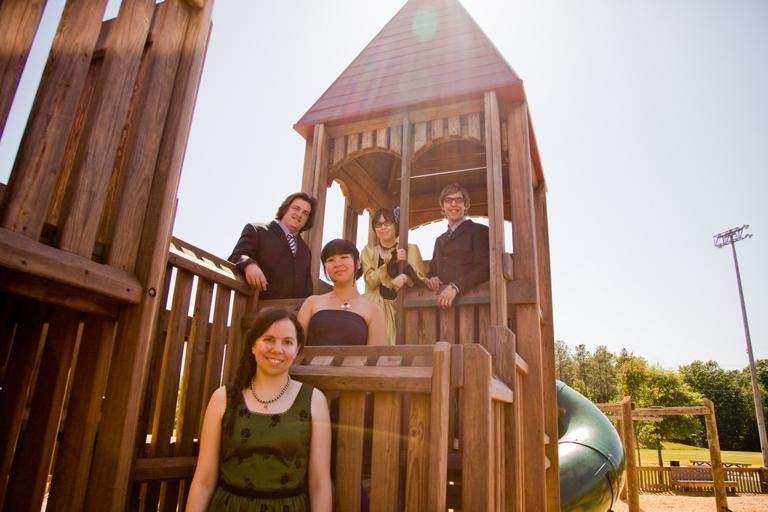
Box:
[668,466,737,494]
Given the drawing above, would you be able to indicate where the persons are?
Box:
[424,183,489,308]
[186,306,334,511]
[296,240,386,347]
[228,192,316,299]
[359,206,424,346]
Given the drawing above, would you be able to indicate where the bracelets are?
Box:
[450,282,461,295]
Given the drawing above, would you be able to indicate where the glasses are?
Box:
[373,218,393,229]
[444,196,465,206]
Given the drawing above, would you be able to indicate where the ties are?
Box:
[286,231,298,257]
[446,228,452,239]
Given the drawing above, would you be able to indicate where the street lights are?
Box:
[714,223,768,469]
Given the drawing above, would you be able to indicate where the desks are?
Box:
[689,459,752,468]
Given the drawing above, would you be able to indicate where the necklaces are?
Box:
[248,375,289,407]
[333,290,359,309]
[379,242,397,251]
